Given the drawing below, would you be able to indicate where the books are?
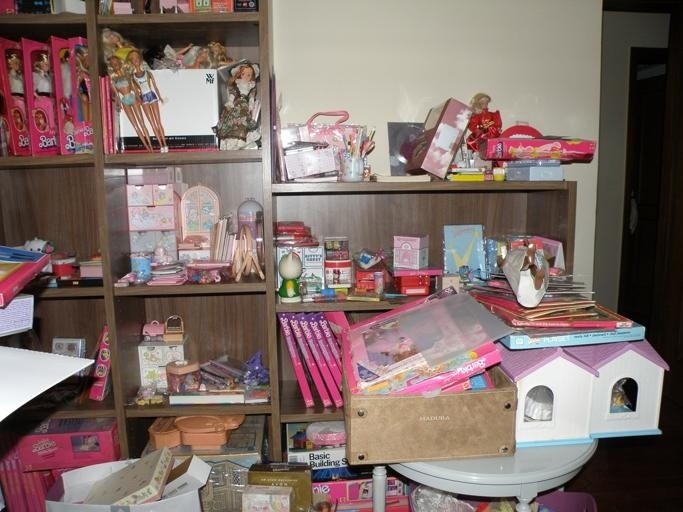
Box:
[469,270,599,321]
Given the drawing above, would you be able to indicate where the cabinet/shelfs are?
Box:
[272,180,579,462]
[0,0,283,468]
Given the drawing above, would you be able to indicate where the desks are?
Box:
[372,438,600,512]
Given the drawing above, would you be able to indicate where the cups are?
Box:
[339,153,366,182]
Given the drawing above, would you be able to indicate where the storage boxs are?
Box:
[0,418,121,512]
[338,327,518,465]
[44,455,205,512]
[285,422,374,481]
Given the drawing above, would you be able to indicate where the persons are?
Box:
[0,28,261,156]
[466,93,501,152]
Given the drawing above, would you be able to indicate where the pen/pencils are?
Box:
[343,125,376,158]
[313,294,381,302]
[447,168,483,172]
[385,294,409,297]
